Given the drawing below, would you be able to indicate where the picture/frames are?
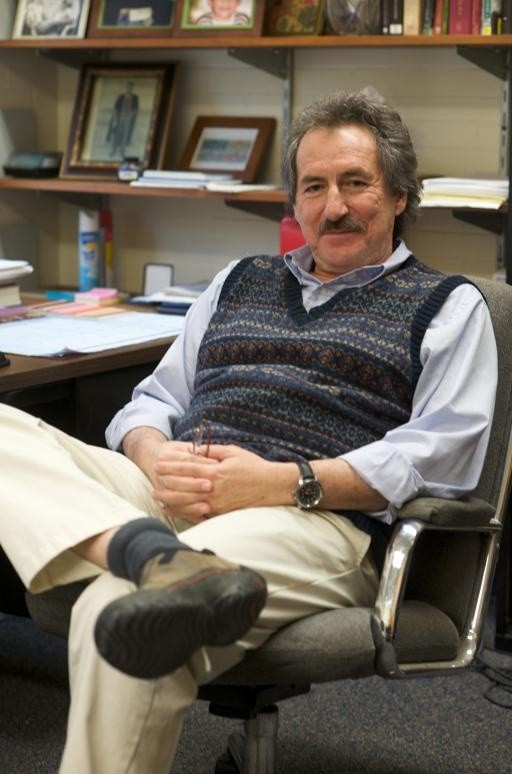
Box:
[10,0,271,43]
[181,115,276,183]
[57,60,186,185]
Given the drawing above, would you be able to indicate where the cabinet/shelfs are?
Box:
[1,31,508,215]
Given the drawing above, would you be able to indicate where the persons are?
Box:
[104,79,141,159]
[196,0,249,24]
[24,0,78,36]
[0,85,499,774]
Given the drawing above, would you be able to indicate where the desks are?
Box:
[0,282,200,393]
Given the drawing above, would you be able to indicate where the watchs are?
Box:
[295,461,323,511]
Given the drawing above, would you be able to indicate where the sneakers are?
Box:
[93,549,268,677]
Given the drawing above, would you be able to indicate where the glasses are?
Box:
[193,418,211,457]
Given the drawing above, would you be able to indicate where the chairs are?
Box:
[199,271,511,774]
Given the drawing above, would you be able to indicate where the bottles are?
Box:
[77,206,115,292]
[118,155,143,180]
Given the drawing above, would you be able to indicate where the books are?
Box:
[383,0,510,34]
